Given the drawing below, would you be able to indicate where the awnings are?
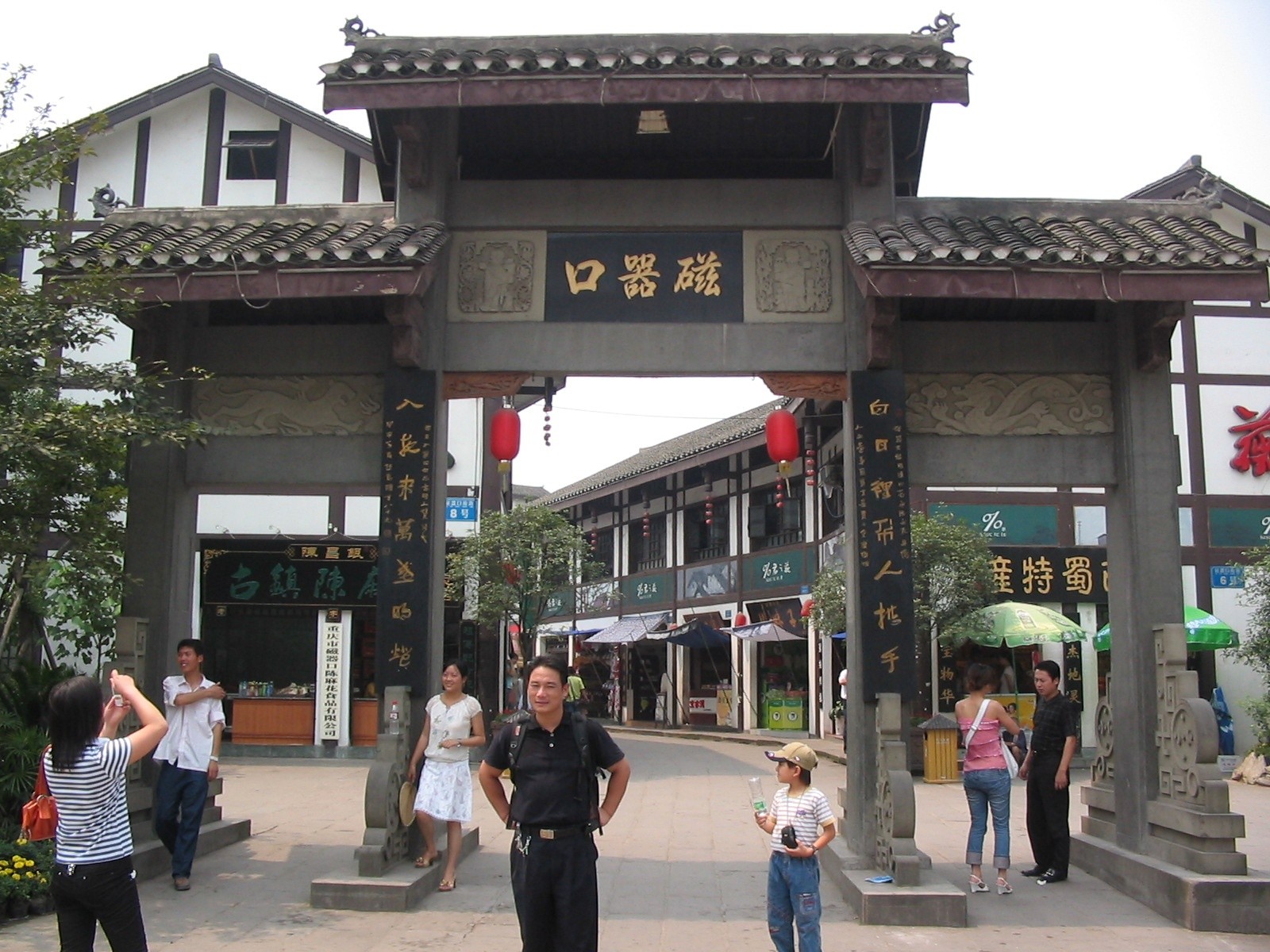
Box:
[584,611,808,657]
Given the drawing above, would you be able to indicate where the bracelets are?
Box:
[456,738,462,747]
[209,756,219,762]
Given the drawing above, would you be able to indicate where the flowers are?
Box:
[0,837,56,903]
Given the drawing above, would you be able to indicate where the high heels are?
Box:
[416,856,435,867]
[968,875,989,892]
[439,874,457,891]
[996,876,1013,895]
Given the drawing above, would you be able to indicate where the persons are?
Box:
[953,662,1022,895]
[41,668,168,952]
[408,658,488,892]
[505,651,529,713]
[562,666,590,711]
[1002,715,1028,767]
[752,740,836,952]
[478,656,630,952]
[838,661,848,754]
[153,637,229,890]
[1012,659,1077,883]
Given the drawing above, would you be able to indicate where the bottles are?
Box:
[389,700,400,735]
[238,679,274,697]
[748,776,768,818]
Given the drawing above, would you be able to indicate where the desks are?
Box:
[225,693,315,745]
[350,696,380,744]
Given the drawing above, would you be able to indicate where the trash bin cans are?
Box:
[917,715,961,784]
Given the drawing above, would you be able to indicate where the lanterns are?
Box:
[490,402,523,477]
[543,404,551,445]
[588,449,818,550]
[735,611,745,628]
[766,406,798,467]
[800,599,814,618]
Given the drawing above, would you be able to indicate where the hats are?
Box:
[765,741,818,772]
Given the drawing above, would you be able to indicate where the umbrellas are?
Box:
[1208,689,1235,755]
[941,598,1089,730]
[1090,603,1241,653]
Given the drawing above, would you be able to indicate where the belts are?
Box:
[522,825,584,839]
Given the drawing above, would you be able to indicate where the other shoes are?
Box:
[1021,866,1045,876]
[175,877,190,890]
[1038,868,1066,882]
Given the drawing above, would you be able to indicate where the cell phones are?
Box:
[112,686,123,707]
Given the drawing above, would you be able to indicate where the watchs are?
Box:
[809,845,819,855]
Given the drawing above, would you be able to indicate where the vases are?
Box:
[0,891,56,918]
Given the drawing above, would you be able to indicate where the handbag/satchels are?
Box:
[22,746,59,842]
[999,737,1019,779]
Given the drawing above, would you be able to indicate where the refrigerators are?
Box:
[964,693,1037,733]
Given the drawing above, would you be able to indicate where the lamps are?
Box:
[327,523,340,532]
[215,525,237,539]
[268,525,295,541]
